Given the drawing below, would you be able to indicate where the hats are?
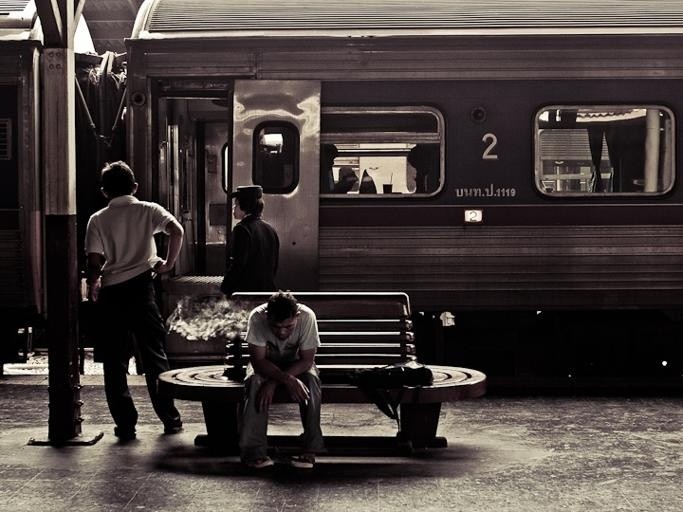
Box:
[231,185,263,197]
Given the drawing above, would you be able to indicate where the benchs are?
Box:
[156,289,489,458]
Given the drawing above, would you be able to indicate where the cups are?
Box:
[383,184,392,194]
[577,182,592,192]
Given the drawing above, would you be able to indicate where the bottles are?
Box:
[551,160,569,193]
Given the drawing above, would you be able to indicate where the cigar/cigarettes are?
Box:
[304,398,308,406]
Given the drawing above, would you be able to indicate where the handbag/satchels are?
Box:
[353,360,432,386]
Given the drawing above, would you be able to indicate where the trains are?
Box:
[0,0,683,400]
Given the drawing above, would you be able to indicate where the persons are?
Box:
[239,291,326,470]
[322,143,338,193]
[333,166,357,194]
[216,185,281,309]
[84,159,183,444]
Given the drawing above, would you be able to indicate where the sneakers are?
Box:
[244,455,275,468]
[116,429,136,443]
[290,454,315,469]
[164,422,182,433]
